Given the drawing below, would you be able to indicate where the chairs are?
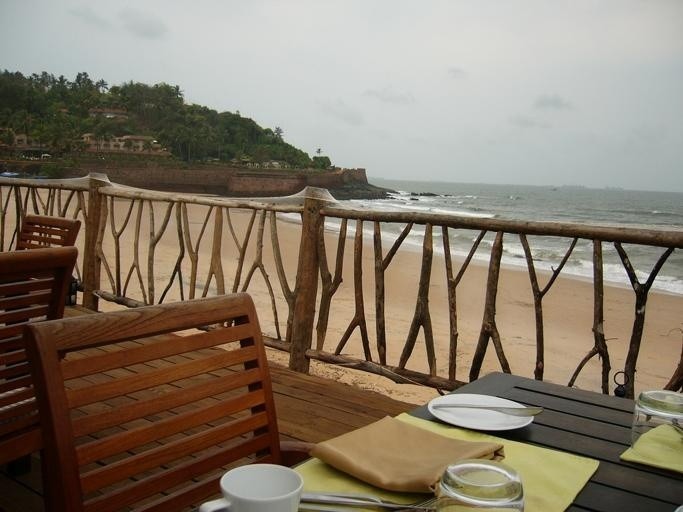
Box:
[22,291,319,512]
[13,212,83,251]
[0,246,81,466]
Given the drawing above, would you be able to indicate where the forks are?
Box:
[301,489,433,511]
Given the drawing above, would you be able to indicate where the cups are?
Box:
[435,458,525,512]
[632,391,683,461]
[199,462,305,512]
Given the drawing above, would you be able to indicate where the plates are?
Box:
[427,393,534,433]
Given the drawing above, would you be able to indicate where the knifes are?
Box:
[433,400,545,418]
